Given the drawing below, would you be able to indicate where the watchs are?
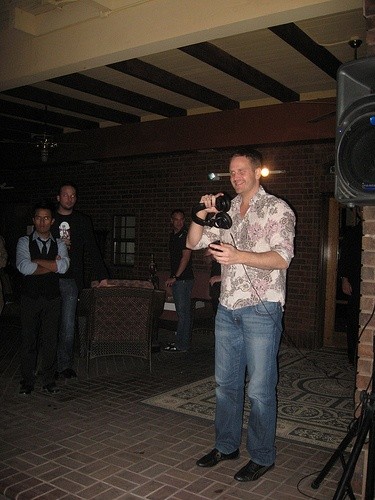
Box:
[172,274,178,280]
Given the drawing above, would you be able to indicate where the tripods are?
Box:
[311,334,375,500]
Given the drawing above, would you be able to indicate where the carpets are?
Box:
[140,347,357,457]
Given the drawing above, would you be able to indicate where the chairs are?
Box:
[77,287,167,379]
[0,264,22,329]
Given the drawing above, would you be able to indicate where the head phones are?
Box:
[191,192,232,229]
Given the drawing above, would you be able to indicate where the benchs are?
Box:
[155,270,212,332]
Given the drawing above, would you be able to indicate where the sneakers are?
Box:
[196,448,239,467]
[234,460,275,482]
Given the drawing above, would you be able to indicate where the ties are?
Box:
[37,236,50,255]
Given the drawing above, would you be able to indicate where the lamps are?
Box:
[33,103,58,162]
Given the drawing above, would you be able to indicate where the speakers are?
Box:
[334,56,375,206]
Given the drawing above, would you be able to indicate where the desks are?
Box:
[91,279,155,289]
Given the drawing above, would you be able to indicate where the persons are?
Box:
[164,148,296,482]
[338,207,363,371]
[0,182,99,397]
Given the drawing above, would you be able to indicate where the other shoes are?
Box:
[42,384,61,394]
[164,342,192,352]
[19,384,34,395]
